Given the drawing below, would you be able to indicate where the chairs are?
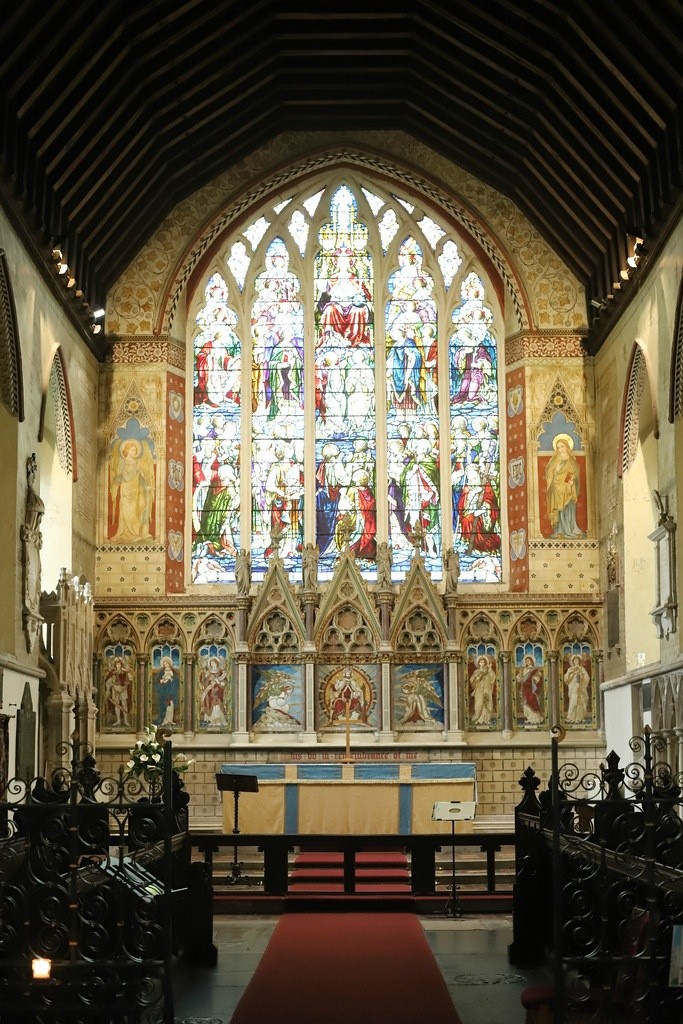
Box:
[521,908,652,1024]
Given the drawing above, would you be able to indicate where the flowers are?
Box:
[123,723,197,785]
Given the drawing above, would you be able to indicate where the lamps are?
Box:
[52,250,106,334]
[590,241,643,309]
